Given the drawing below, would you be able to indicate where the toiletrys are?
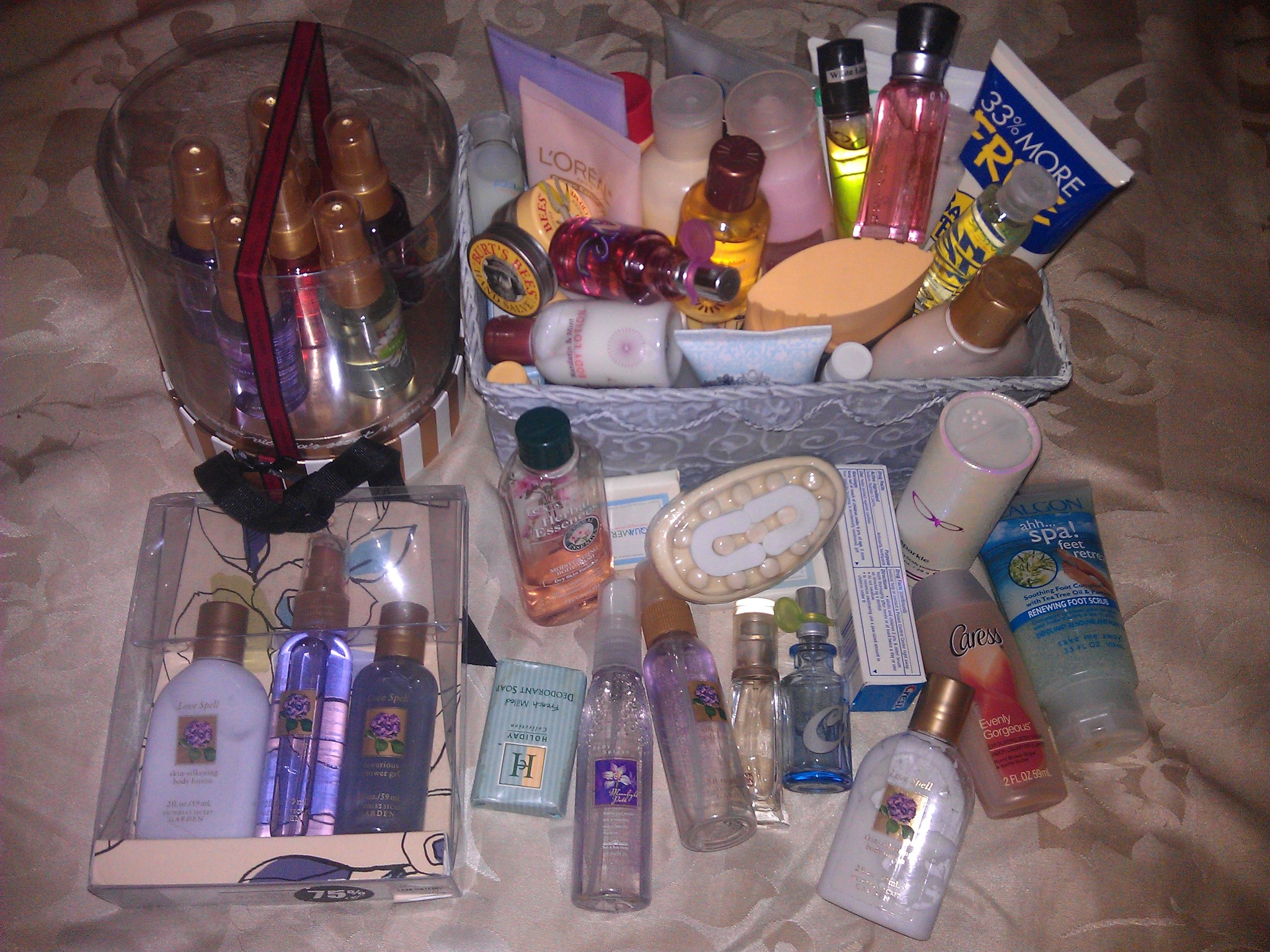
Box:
[88,3,1151,943]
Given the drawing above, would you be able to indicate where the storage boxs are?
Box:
[457,108,1075,496]
[98,18,467,499]
[84,476,469,913]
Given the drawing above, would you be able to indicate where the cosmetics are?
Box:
[773,584,856,797]
[912,566,1070,821]
[495,403,615,626]
[163,77,430,426]
[458,2,1133,385]
[893,402,1044,578]
[730,609,791,831]
[830,467,927,718]
[814,668,982,939]
[974,473,1151,764]
[631,556,760,849]
[134,533,439,836]
[566,573,655,912]
